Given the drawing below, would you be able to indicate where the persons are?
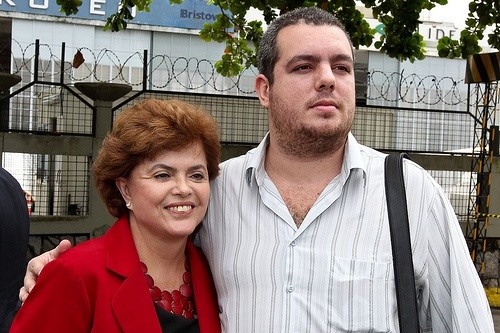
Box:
[20,7,495,333]
[7,101,223,333]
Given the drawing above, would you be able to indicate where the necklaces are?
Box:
[140,257,199,321]
